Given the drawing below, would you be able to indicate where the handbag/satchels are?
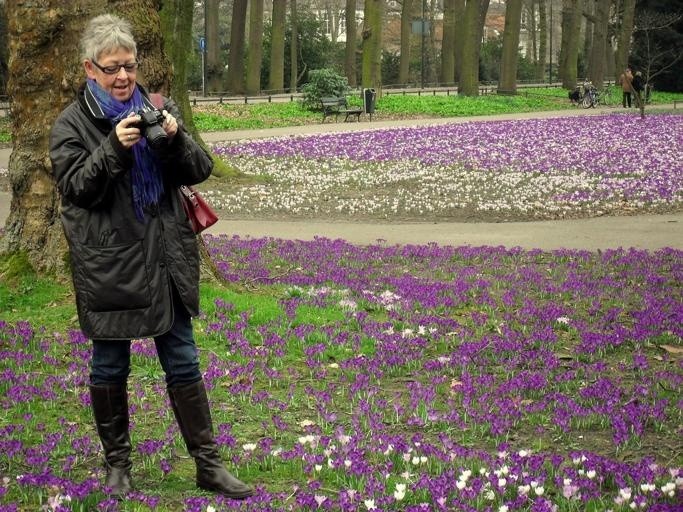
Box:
[147,92,218,235]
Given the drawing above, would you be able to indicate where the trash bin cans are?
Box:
[363,88,376,122]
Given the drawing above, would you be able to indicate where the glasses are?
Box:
[92,58,140,74]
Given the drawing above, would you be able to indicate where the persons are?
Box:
[619,68,644,108]
[50,13,253,499]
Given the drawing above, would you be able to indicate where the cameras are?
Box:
[126,108,170,151]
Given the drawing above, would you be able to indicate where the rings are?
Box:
[128,135,131,141]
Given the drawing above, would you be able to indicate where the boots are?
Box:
[167,375,252,499]
[89,383,133,499]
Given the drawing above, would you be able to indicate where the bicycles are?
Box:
[568,81,618,109]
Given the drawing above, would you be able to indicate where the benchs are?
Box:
[320,97,364,123]
